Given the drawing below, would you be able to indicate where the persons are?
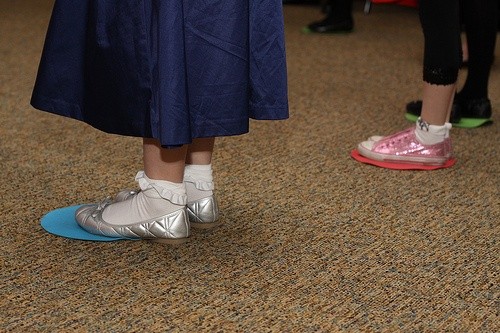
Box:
[356,0,464,166]
[29,0,290,246]
[306,0,354,33]
[405,0,500,123]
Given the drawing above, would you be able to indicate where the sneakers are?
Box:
[405,94,492,127]
[357,130,454,165]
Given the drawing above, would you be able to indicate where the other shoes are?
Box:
[304,19,353,33]
[116,190,219,228]
[75,200,190,244]
